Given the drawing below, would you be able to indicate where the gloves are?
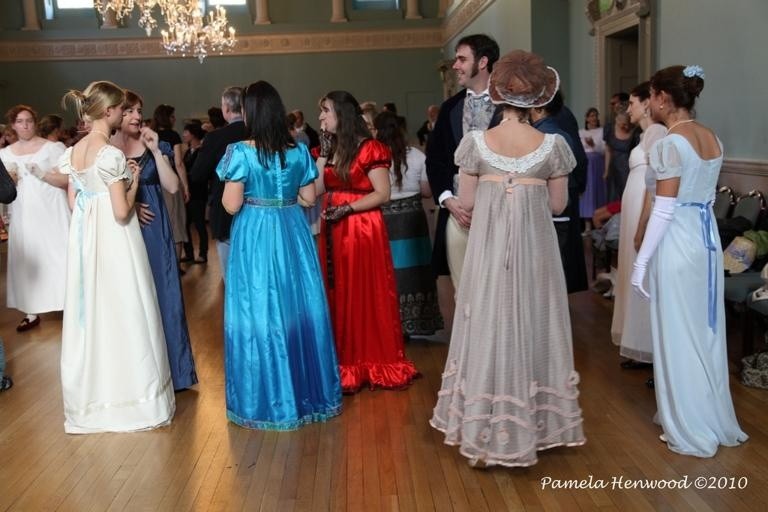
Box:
[630,193,677,299]
[320,200,353,222]
[318,128,335,158]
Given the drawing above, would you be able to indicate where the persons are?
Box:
[0,35,748,470]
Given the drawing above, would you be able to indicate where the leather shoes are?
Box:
[15,314,40,332]
[646,378,655,389]
[0,374,12,394]
[620,356,641,370]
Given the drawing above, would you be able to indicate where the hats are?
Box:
[486,49,561,110]
[720,234,757,276]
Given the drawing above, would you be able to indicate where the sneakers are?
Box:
[178,255,194,262]
[192,257,208,264]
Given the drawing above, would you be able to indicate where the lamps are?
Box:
[92,0,235,64]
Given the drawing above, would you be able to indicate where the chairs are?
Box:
[727,190,766,233]
[711,186,734,224]
[743,286,768,365]
[721,266,762,362]
